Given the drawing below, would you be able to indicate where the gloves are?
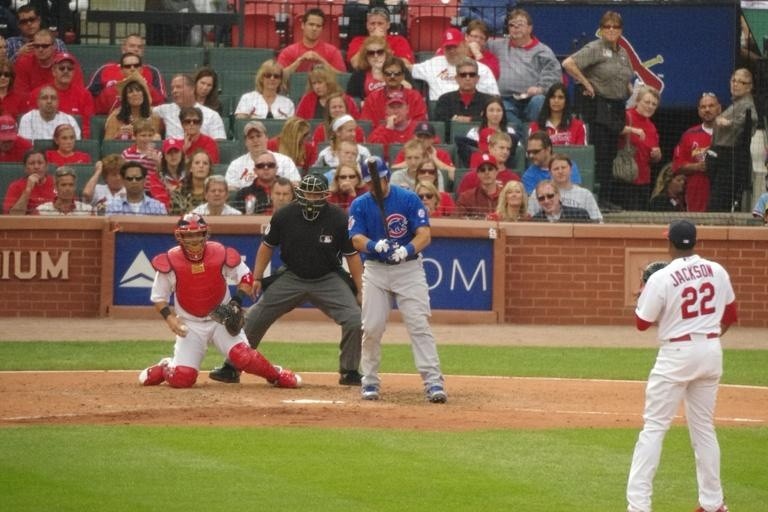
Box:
[366,238,415,265]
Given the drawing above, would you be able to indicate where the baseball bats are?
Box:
[368,157,398,254]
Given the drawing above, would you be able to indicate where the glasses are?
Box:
[731,78,750,85]
[478,167,494,172]
[527,148,544,154]
[419,168,436,174]
[182,118,201,125]
[0,71,11,77]
[538,193,556,201]
[457,72,477,78]
[419,192,433,199]
[123,62,141,69]
[602,24,623,30]
[32,43,52,48]
[264,73,282,79]
[18,16,37,24]
[509,21,526,28]
[254,162,276,168]
[383,71,403,76]
[58,64,74,71]
[338,174,357,179]
[125,173,145,181]
[366,48,386,57]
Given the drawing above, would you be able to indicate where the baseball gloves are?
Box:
[639,261,668,284]
[212,301,248,336]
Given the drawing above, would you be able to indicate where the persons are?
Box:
[208,172,365,386]
[627,220,738,512]
[348,156,447,403]
[139,213,302,388]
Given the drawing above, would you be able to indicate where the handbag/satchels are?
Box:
[612,111,638,182]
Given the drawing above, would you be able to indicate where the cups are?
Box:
[693,153,706,162]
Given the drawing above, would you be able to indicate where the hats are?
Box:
[162,137,183,154]
[475,153,499,174]
[441,28,465,48]
[361,155,392,183]
[663,220,697,246]
[113,70,152,104]
[414,121,436,138]
[0,114,18,142]
[385,90,408,105]
[52,52,77,65]
[244,120,267,137]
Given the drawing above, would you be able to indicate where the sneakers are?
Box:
[138,357,174,387]
[208,363,241,383]
[267,365,302,389]
[427,386,447,403]
[339,370,364,386]
[361,384,380,401]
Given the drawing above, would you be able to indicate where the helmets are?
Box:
[174,212,209,263]
[292,174,332,222]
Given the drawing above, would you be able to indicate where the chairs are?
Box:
[0,2,596,219]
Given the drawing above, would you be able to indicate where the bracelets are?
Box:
[253,272,263,281]
[237,289,247,301]
[366,240,377,254]
[404,242,416,256]
[159,307,171,320]
